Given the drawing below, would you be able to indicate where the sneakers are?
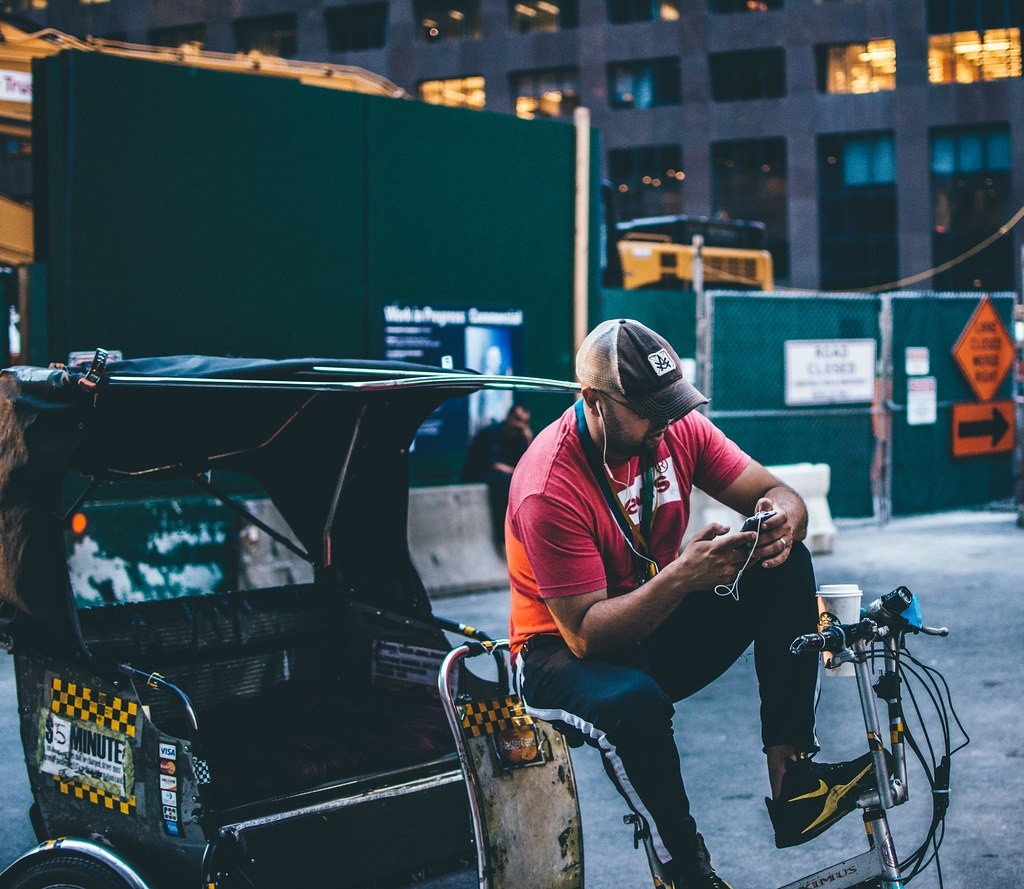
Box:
[648,867,733,889]
[764,747,893,849]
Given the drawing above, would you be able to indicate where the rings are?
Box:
[780,538,787,549]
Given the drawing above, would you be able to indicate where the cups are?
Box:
[816,584,863,676]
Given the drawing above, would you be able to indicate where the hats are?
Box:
[575,318,709,426]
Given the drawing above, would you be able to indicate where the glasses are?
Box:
[512,411,529,424]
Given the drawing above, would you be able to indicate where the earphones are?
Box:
[595,400,602,415]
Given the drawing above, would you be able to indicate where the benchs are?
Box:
[78,583,475,889]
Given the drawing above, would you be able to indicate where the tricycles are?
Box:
[0,346,970,889]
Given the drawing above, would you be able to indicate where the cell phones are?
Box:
[741,510,778,533]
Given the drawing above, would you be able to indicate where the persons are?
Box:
[488,403,533,561]
[504,320,893,889]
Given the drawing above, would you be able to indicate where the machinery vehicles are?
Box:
[0,24,775,294]
